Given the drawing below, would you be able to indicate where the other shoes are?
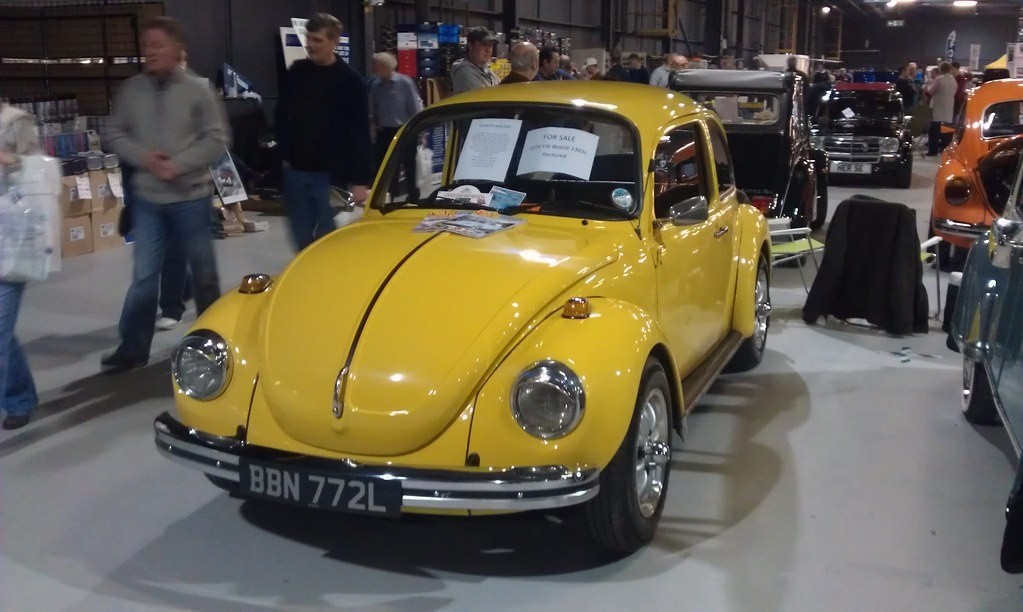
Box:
[3,416,26,428]
[155,316,183,331]
[103,343,148,374]
[925,152,937,156]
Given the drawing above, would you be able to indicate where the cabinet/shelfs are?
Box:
[0,0,166,116]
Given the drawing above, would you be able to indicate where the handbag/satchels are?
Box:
[0,153,60,283]
[415,139,434,190]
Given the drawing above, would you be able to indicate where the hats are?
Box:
[467,26,498,43]
[582,57,597,67]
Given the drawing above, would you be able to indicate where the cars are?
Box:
[661,69,827,268]
[849,69,919,110]
[931,78,1023,270]
[151,79,772,558]
[941,147,1023,574]
[808,80,914,190]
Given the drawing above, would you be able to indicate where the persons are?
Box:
[531,46,576,81]
[270,14,374,252]
[810,64,852,124]
[366,51,426,204]
[745,54,768,102]
[649,52,679,87]
[627,54,651,83]
[782,56,810,104]
[667,55,688,72]
[0,93,37,429]
[603,49,630,82]
[99,15,230,368]
[580,57,604,81]
[896,61,976,157]
[450,25,501,95]
[496,41,540,85]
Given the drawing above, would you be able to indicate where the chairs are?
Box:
[905,106,934,158]
[837,98,857,117]
[920,234,944,319]
[765,216,826,292]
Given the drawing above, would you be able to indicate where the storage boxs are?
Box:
[59,167,126,256]
